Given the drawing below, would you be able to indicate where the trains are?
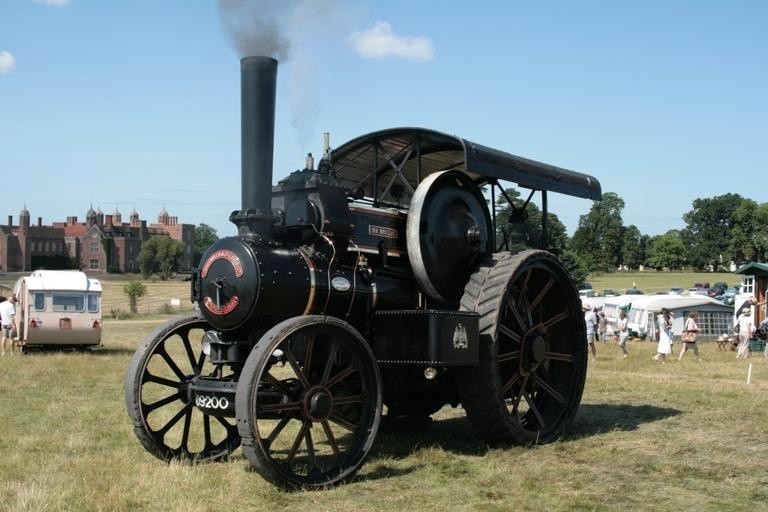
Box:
[125,57,602,491]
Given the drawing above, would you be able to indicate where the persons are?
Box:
[677,311,703,361]
[598,312,607,343]
[617,310,629,361]
[590,306,598,342]
[581,303,596,362]
[732,295,758,342]
[665,314,673,356]
[0,294,18,356]
[735,307,752,360]
[650,310,672,362]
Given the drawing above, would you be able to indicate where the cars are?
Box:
[579,287,643,297]
[660,280,740,303]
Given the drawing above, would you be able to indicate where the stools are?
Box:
[715,340,728,352]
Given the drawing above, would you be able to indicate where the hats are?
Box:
[583,304,591,311]
[10,294,18,302]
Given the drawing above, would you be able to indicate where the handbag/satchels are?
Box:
[681,329,697,343]
